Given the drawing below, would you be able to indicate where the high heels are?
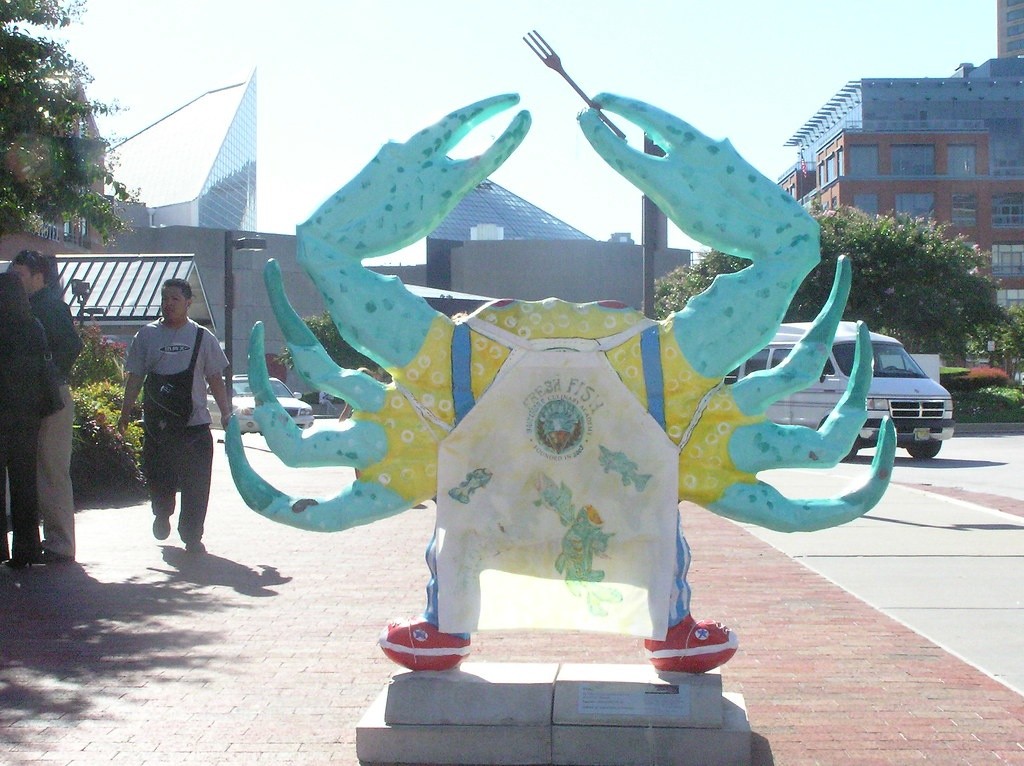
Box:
[6,556,34,569]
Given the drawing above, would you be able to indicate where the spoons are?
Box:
[522,30,627,140]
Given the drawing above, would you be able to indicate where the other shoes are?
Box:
[35,549,74,563]
[185,540,205,553]
[152,516,171,540]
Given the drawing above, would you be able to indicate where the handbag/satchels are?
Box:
[145,369,193,414]
[36,318,65,418]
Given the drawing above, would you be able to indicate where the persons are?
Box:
[319,367,380,479]
[118,279,232,554]
[0,250,85,570]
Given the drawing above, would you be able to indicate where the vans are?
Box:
[724,322,957,464]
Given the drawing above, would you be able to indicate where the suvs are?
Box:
[204,372,314,436]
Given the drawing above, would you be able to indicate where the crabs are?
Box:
[220,90,900,673]
